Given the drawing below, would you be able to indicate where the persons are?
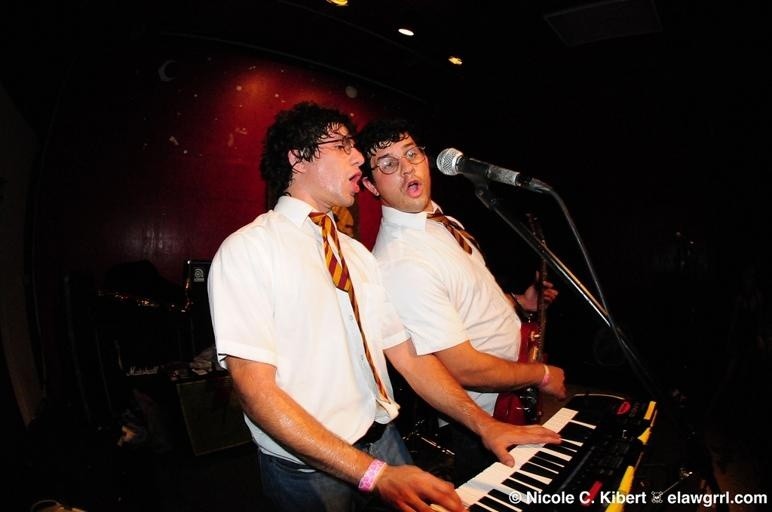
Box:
[704,262,772,418]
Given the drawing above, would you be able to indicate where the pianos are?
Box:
[429,393,659,512]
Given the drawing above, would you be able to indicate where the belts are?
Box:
[354,423,387,450]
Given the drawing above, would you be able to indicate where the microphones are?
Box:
[434,147,554,195]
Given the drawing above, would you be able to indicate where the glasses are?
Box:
[309,136,358,156]
[371,145,426,175]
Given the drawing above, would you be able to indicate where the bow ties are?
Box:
[428,210,481,255]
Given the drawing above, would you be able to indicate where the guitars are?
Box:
[493,212,547,425]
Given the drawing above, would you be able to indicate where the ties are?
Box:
[309,211,392,404]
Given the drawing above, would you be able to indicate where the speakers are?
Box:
[175,372,253,457]
